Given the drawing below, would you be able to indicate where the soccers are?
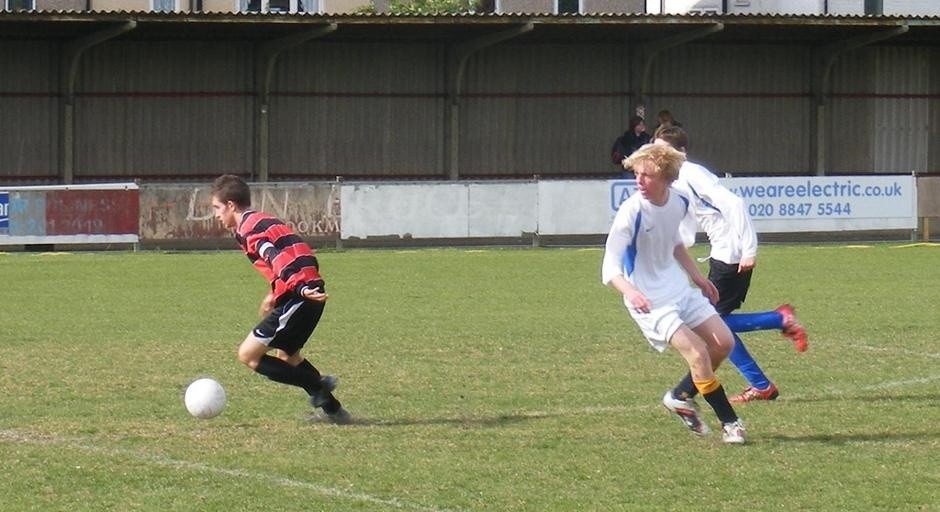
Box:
[185,378,226,419]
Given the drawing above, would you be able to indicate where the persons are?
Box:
[602,145,745,444]
[654,127,808,402]
[654,110,683,131]
[612,115,652,178]
[211,175,352,423]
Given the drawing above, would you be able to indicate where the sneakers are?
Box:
[727,381,780,402]
[720,417,746,446]
[661,389,715,437]
[772,302,809,354]
[305,405,354,425]
[309,373,338,409]
